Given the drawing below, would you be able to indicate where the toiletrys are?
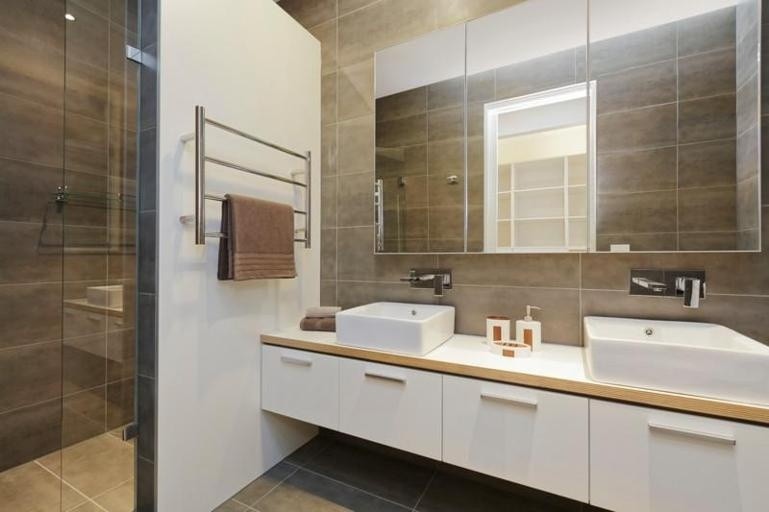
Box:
[515,304,543,353]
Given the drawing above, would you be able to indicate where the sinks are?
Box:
[86,284,123,308]
[582,316,769,406]
[334,301,455,358]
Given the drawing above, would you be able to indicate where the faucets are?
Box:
[399,270,444,298]
[674,276,702,310]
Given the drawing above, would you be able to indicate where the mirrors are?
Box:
[373,0,762,256]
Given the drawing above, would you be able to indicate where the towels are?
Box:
[299,304,341,332]
[215,192,299,280]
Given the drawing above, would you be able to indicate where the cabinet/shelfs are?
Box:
[441,374,587,500]
[256,340,335,428]
[83,313,107,360]
[62,308,83,348]
[589,401,769,512]
[107,317,128,365]
[337,357,441,466]
